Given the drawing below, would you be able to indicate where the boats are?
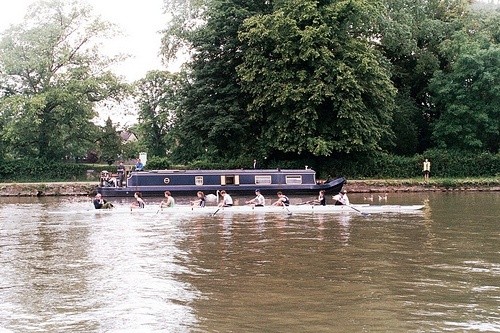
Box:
[73,203,425,215]
[95,164,346,197]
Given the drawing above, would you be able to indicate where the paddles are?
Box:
[212,206,224,216]
[346,205,371,216]
[250,204,256,214]
[156,204,164,214]
[311,206,316,215]
[282,203,293,215]
[130,207,132,215]
[296,200,313,206]
[191,206,194,214]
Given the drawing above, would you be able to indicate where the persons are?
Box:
[335,192,347,205]
[161,191,174,208]
[94,193,113,209]
[221,190,233,207]
[197,191,208,206]
[245,189,265,207]
[422,159,431,181]
[313,190,326,206]
[276,192,290,206]
[136,159,144,171]
[117,161,126,187]
[105,178,115,187]
[131,192,145,209]
